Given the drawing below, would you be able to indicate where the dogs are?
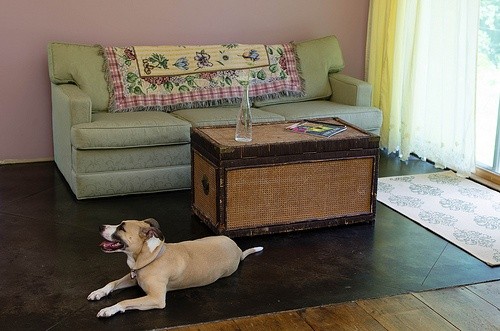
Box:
[87,217,264,318]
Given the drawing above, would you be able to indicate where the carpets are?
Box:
[374,168,500,269]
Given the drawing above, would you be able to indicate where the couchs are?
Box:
[45,33,384,200]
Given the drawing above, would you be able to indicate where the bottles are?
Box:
[235,81,252,143]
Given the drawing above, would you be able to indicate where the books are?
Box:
[284,119,348,139]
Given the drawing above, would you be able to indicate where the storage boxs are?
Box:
[188,115,382,240]
